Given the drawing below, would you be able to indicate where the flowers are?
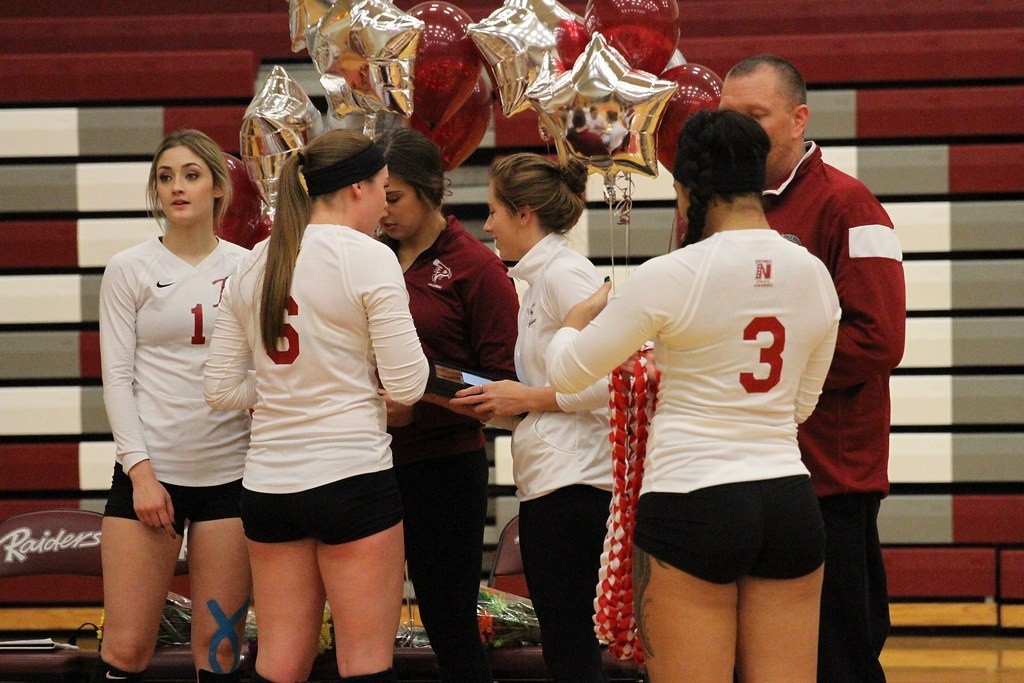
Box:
[96,591,258,647]
[478,583,542,650]
[317,601,334,655]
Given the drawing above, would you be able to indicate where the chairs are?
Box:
[0,508,647,683]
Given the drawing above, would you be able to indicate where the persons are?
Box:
[718,53,906,683]
[449,152,655,683]
[99,129,254,683]
[545,109,842,683]
[204,126,430,683]
[373,126,520,683]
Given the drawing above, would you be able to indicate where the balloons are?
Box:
[215,0,724,250]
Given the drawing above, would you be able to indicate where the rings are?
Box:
[480,384,483,394]
